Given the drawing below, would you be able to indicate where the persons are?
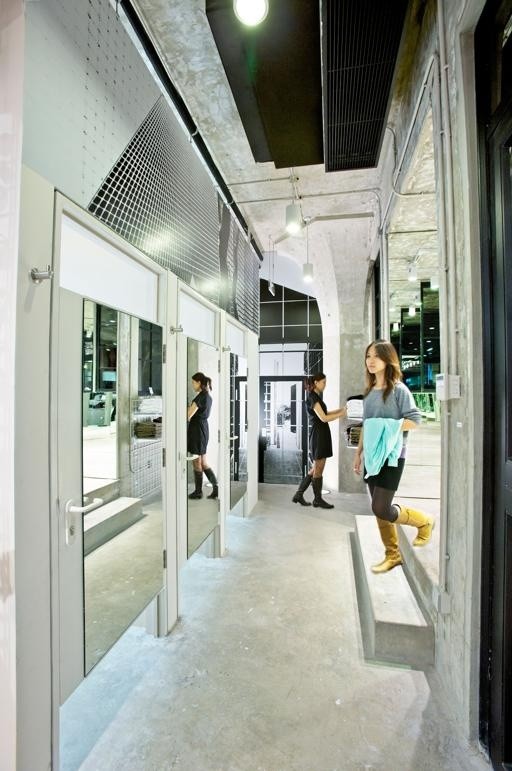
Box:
[354,341,435,573]
[187,373,218,498]
[292,372,346,508]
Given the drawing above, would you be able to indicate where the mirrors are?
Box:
[229,352,250,511]
[81,297,165,674]
[185,334,225,561]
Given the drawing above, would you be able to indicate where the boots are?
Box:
[372,515,403,573]
[204,469,218,499]
[392,504,436,546]
[311,477,334,508]
[188,470,203,499]
[292,475,312,506]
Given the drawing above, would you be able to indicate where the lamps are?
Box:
[287,173,318,286]
